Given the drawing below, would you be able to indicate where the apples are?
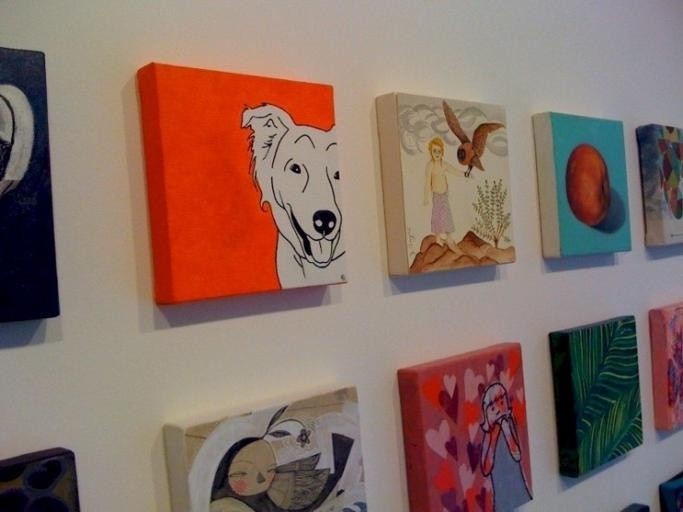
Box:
[566,144,610,225]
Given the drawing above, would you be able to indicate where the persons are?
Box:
[423,139,474,246]
